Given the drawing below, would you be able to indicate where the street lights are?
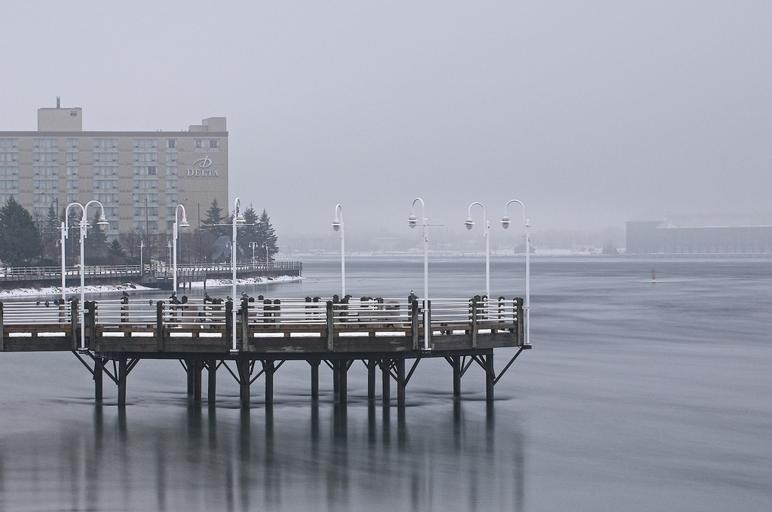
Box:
[407,196,430,350]
[248,241,259,268]
[140,239,146,277]
[261,241,269,268]
[78,199,109,351]
[60,202,92,300]
[465,201,490,320]
[166,239,173,271]
[500,198,531,347]
[331,203,346,299]
[229,195,246,354]
[172,202,190,298]
[226,241,233,270]
